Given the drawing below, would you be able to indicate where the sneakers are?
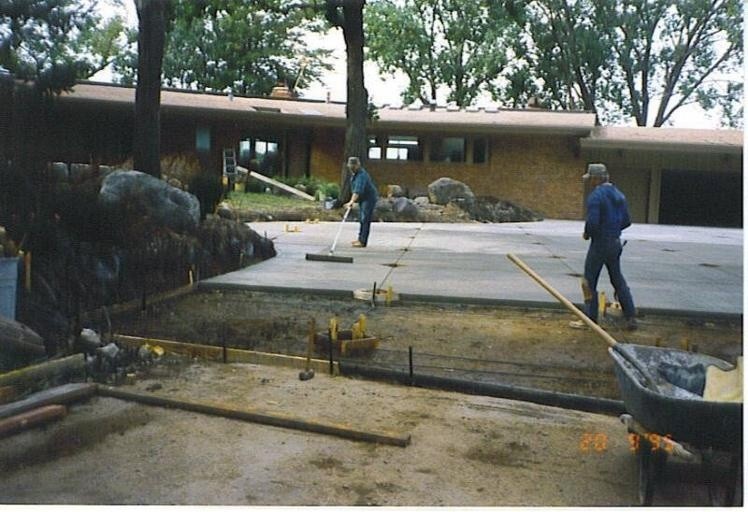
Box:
[625,318,640,329]
[569,319,595,329]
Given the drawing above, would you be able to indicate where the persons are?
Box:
[344,157,379,247]
[569,163,637,330]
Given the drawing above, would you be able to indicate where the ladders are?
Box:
[223,145,238,176]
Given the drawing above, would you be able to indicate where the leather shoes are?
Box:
[353,241,364,247]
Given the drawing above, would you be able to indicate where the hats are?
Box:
[346,156,360,167]
[581,163,610,177]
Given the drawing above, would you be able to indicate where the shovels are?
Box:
[506,252,704,403]
